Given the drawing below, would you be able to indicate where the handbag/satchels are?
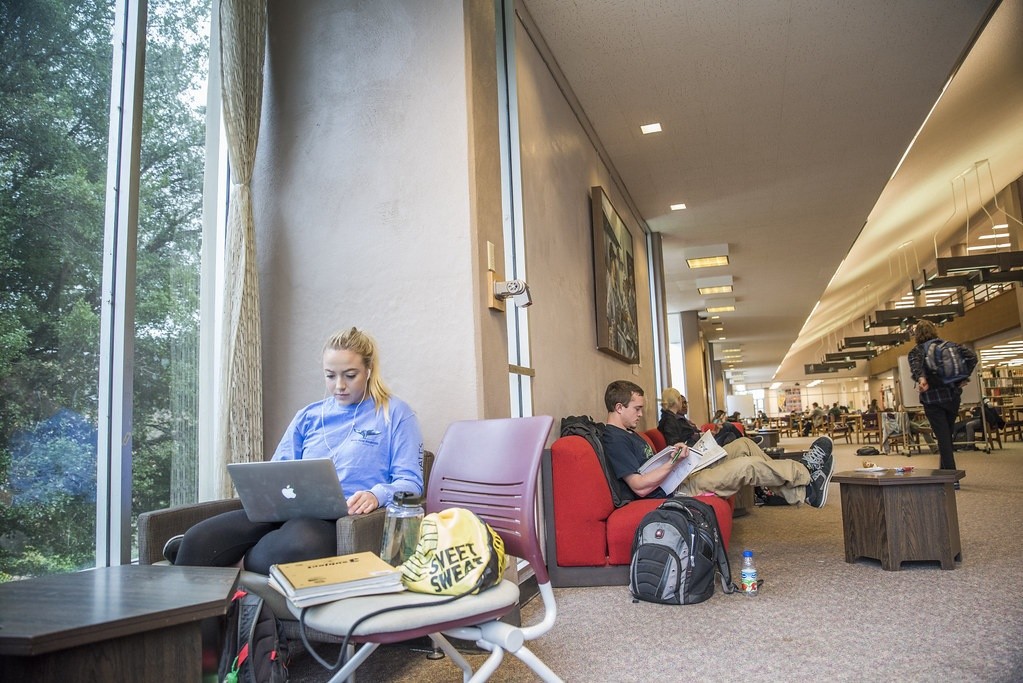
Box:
[396,507,505,595]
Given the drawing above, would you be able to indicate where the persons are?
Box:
[907,411,939,454]
[676,395,764,447]
[831,403,841,422]
[712,410,724,423]
[729,412,741,422]
[658,388,766,506]
[603,380,834,509]
[757,411,768,421]
[802,403,824,436]
[840,406,854,432]
[908,320,978,490]
[869,399,881,426]
[954,395,1005,450]
[162,327,424,672]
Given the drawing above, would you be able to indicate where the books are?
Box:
[981,369,1023,416]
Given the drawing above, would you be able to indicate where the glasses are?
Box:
[680,401,689,406]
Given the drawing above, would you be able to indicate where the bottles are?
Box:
[741,551,758,598]
[379,490,425,567]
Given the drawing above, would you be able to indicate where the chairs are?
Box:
[756,402,1023,457]
[287,416,566,683]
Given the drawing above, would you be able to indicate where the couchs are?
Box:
[544,422,744,584]
[137,449,434,683]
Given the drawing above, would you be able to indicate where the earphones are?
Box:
[366,368,371,380]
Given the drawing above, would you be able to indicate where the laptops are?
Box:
[226,458,349,522]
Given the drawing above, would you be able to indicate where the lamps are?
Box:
[684,243,730,271]
[802,158,1023,374]
[704,296,739,316]
[720,342,744,354]
[723,350,745,383]
[696,275,733,296]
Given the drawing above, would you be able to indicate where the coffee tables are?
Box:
[761,448,813,461]
[746,431,782,448]
[761,428,780,442]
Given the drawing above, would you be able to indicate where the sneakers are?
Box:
[799,435,834,475]
[804,454,834,508]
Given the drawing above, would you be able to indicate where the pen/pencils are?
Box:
[670,440,689,465]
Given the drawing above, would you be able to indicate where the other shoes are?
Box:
[163,534,184,565]
[963,444,976,450]
[953,480,960,490]
[749,435,764,445]
[753,491,769,506]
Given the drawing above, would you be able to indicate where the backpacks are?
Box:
[918,337,969,385]
[217,586,290,683]
[628,496,739,606]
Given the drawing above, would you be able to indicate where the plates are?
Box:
[855,467,888,472]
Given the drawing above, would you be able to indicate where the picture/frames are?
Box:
[592,185,640,365]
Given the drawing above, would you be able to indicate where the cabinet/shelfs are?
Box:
[980,363,1023,400]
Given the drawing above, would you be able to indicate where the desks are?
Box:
[830,468,967,572]
[0,563,240,683]
